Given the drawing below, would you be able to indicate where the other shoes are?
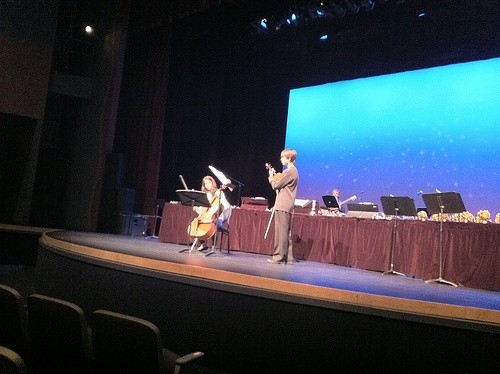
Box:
[267,258,293,265]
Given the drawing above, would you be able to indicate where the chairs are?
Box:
[27,295,92,374]
[0,284,27,364]
[0,345,25,374]
[205,205,232,254]
[119,199,159,238]
[92,310,205,374]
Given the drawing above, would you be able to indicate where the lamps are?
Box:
[251,0,375,36]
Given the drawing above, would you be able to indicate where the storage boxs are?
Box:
[241,197,269,211]
[294,200,319,214]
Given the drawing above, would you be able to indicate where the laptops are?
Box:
[322,195,339,208]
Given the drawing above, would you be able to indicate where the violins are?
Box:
[264,162,283,182]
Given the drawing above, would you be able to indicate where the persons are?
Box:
[331,189,345,212]
[266,149,299,265]
[188,175,230,251]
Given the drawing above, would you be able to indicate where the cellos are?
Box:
[187,183,237,241]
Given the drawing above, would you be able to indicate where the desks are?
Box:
[158,202,500,292]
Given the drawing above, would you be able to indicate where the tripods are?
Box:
[422,192,466,288]
[176,190,211,253]
[145,199,165,239]
[380,196,418,277]
